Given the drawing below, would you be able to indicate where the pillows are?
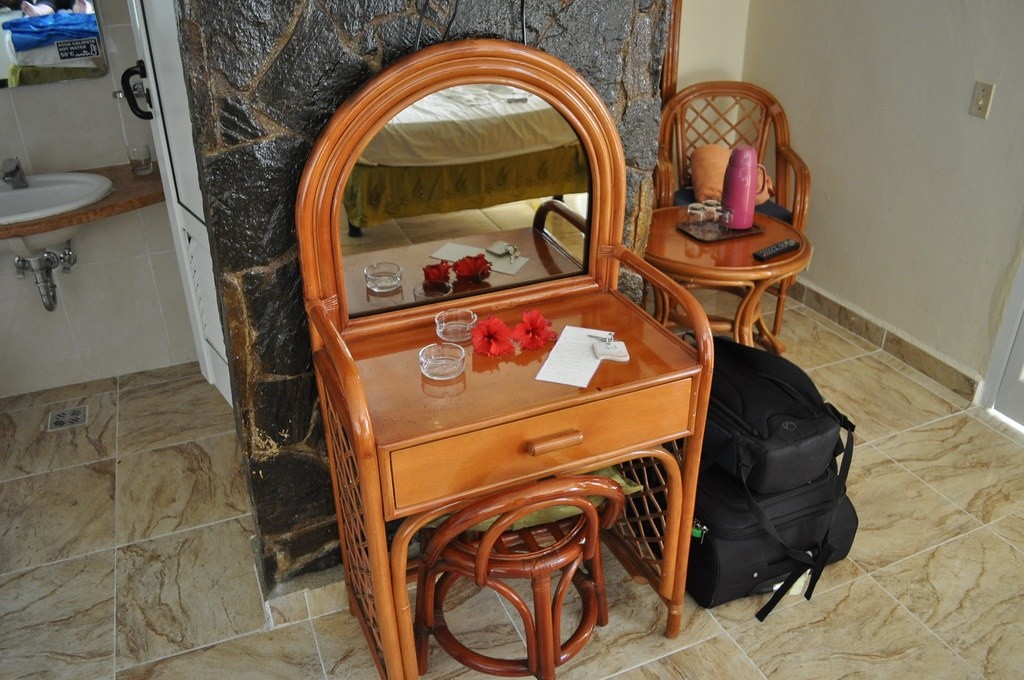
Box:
[423,467,644,532]
[690,143,776,205]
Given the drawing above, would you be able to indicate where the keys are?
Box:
[588,335,612,343]
[509,249,519,264]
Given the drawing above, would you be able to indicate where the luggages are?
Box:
[613,450,859,609]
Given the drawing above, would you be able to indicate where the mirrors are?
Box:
[0,0,107,90]
[337,82,593,320]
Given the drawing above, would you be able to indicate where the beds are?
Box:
[342,84,593,236]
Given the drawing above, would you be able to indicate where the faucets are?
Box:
[0,157,29,189]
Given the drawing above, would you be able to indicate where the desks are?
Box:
[296,38,712,680]
[648,205,813,353]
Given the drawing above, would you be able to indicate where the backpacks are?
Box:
[662,331,856,621]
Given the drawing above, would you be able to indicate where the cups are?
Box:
[720,145,766,229]
[126,143,153,176]
[686,200,733,234]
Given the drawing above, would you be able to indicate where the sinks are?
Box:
[0,172,113,260]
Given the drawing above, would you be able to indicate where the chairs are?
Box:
[643,80,813,334]
[413,473,626,680]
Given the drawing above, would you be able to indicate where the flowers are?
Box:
[422,260,452,294]
[470,314,516,357]
[512,309,558,349]
[452,252,492,280]
